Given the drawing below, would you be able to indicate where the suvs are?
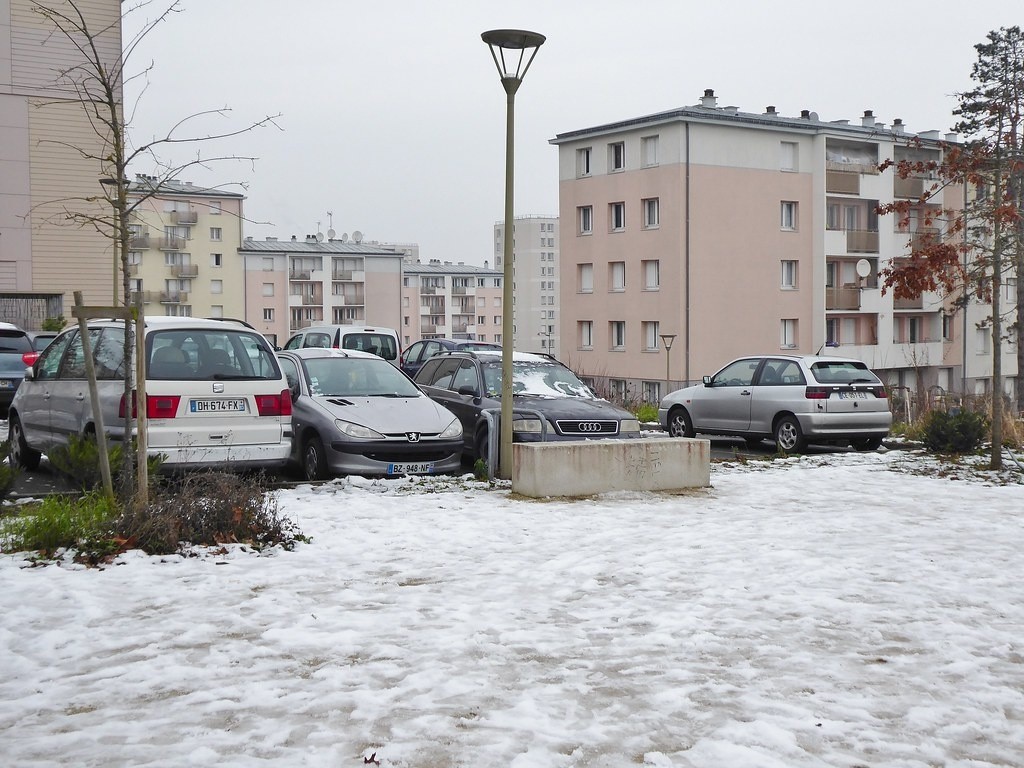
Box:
[409,349,643,480]
[658,354,893,456]
[7,312,296,493]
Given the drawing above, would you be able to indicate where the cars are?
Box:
[275,346,465,483]
[26,330,59,359]
[399,338,506,380]
[0,321,38,408]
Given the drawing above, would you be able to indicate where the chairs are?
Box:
[149,346,243,379]
[346,340,392,360]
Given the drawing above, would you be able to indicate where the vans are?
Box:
[281,322,404,373]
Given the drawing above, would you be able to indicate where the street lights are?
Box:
[479,27,547,478]
[97,177,133,304]
[660,334,679,398]
